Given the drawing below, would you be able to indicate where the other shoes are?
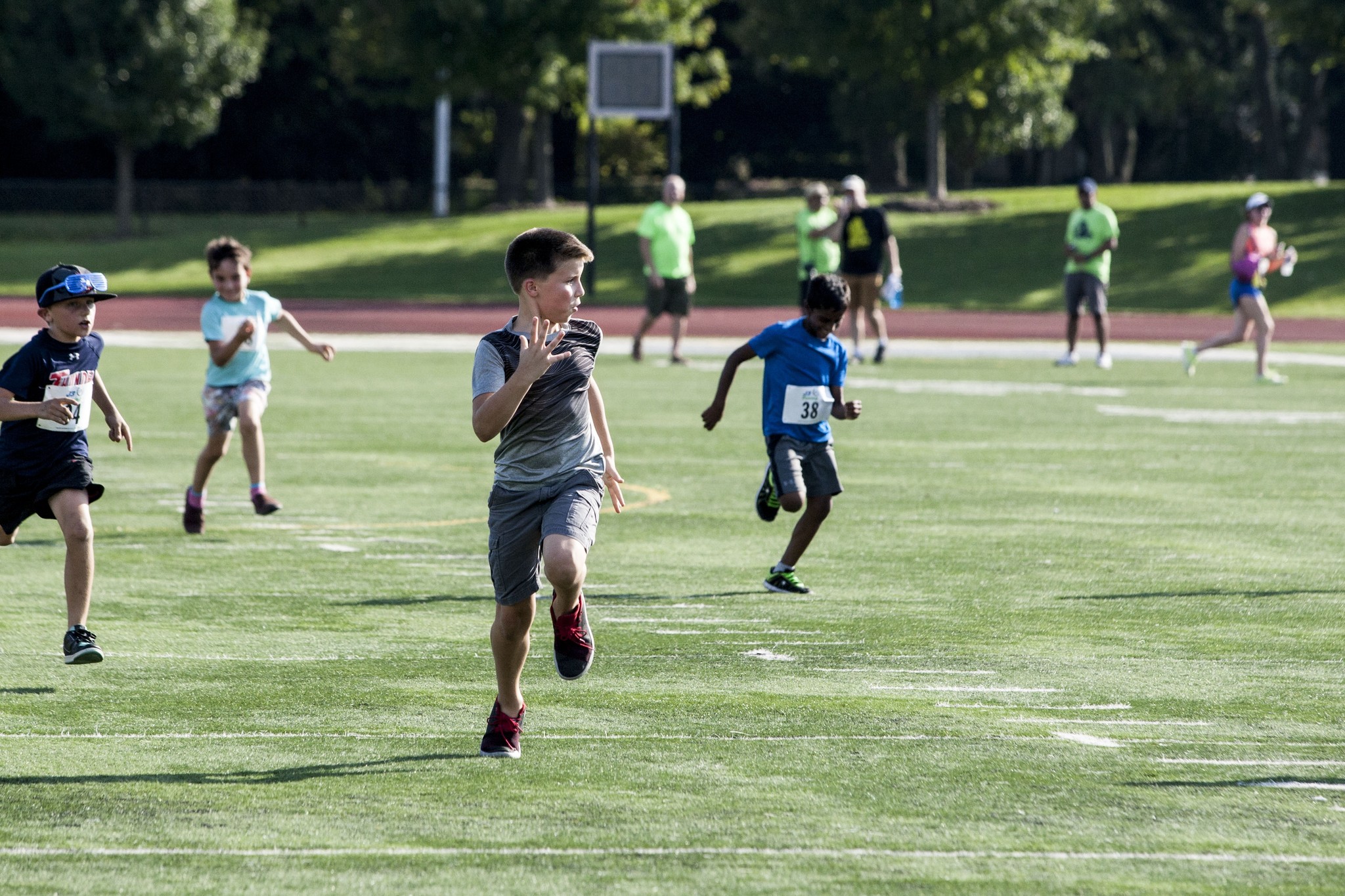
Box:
[1256,367,1289,386]
[1183,348,1198,375]
[1055,351,1079,365]
[633,336,642,361]
[673,355,690,363]
[1095,351,1113,369]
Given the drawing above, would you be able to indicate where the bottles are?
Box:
[1281,245,1297,277]
[887,274,904,310]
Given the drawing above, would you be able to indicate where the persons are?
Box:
[1182,191,1299,387]
[1053,177,1120,370]
[0,261,132,665]
[630,173,697,366]
[181,234,337,536]
[471,227,625,760]
[792,174,904,367]
[700,271,863,595]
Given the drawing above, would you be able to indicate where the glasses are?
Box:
[38,272,107,303]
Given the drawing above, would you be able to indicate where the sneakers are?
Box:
[184,487,204,533]
[252,492,283,515]
[755,461,781,522]
[550,587,595,680]
[480,693,526,758]
[63,624,104,664]
[763,566,810,593]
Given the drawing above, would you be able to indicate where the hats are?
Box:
[841,175,865,193]
[1077,177,1097,192]
[1245,191,1268,214]
[35,264,118,309]
